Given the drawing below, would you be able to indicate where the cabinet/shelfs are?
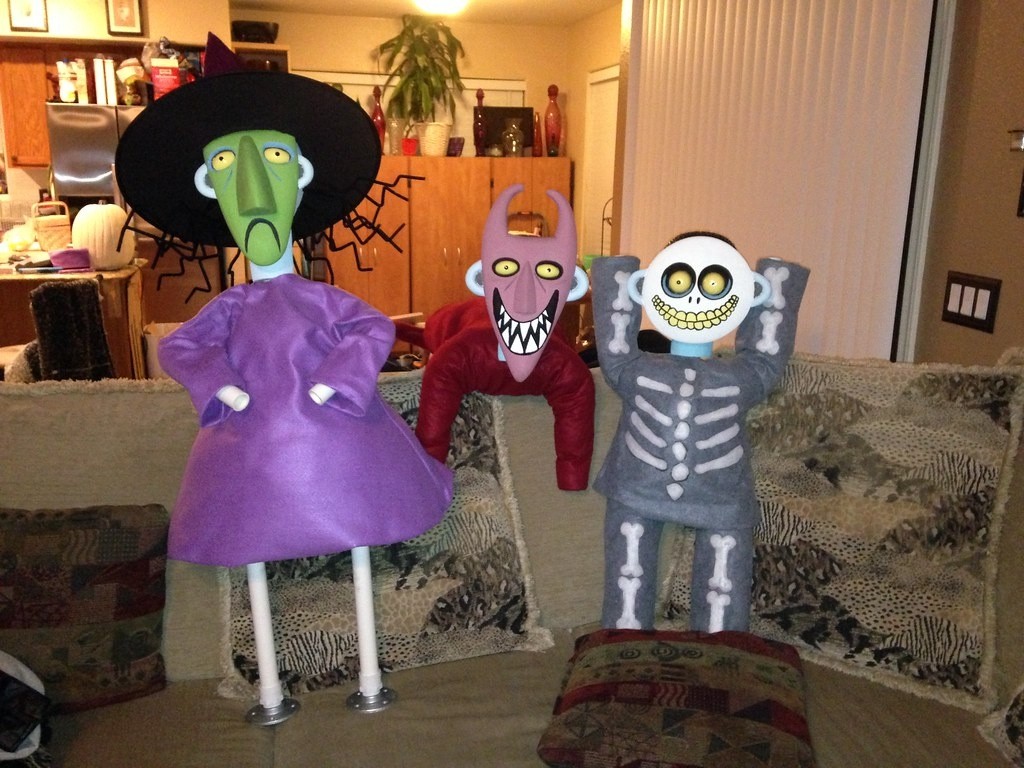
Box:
[293,158,571,360]
[0,41,142,168]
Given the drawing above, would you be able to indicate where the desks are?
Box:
[0,257,148,380]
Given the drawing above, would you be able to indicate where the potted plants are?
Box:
[377,14,465,157]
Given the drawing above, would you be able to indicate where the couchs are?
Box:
[0,349,1024,768]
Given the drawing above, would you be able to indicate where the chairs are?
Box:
[27,277,116,381]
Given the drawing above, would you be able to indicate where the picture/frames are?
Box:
[105,0,144,36]
[7,0,49,33]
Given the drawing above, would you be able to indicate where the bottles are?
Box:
[501,118,525,156]
[532,112,543,157]
[544,84,562,156]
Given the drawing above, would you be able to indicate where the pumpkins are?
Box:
[71,200,134,271]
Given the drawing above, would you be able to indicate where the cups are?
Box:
[387,118,405,156]
[446,137,464,156]
[401,138,418,156]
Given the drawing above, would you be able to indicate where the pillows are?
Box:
[0,504,172,714]
[537,628,817,768]
[650,347,1023,713]
[209,371,552,700]
